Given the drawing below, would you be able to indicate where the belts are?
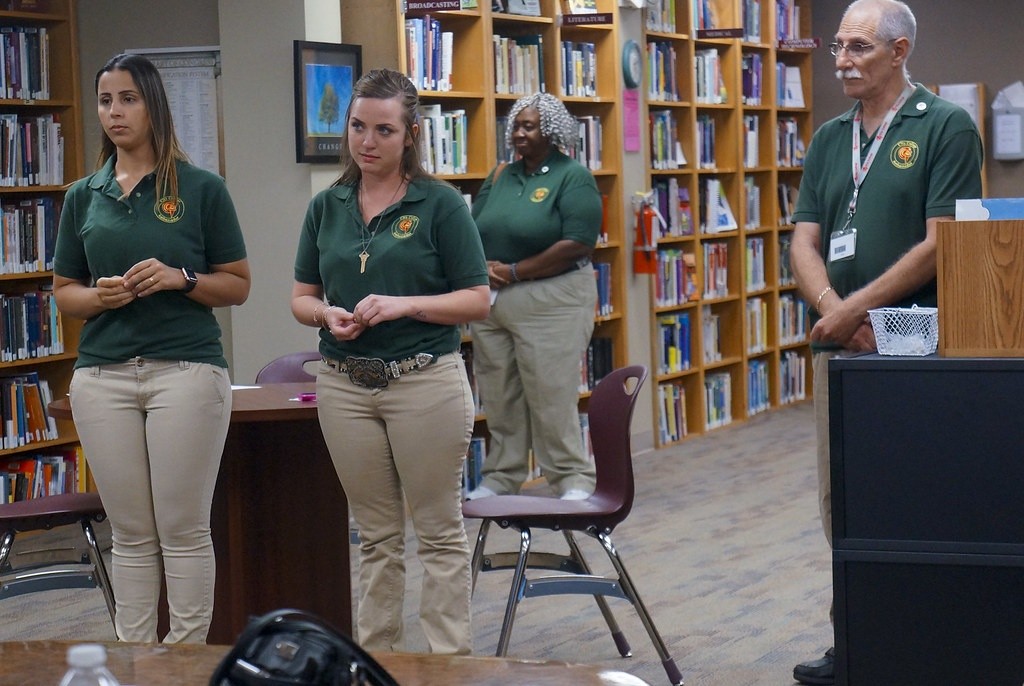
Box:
[568,258,588,270]
[321,353,433,388]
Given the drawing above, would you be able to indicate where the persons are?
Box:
[790,0,984,684]
[291,68,491,657]
[55,54,251,645]
[464,92,603,501]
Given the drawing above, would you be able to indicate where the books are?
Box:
[0,26,87,505]
[403,0,806,493]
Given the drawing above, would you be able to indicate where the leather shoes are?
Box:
[793,647,835,684]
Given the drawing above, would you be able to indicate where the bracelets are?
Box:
[816,286,834,316]
[511,263,520,282]
[313,304,335,332]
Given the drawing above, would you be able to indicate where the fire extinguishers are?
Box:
[630,189,667,274]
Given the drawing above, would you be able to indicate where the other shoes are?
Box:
[465,486,496,501]
[560,489,589,500]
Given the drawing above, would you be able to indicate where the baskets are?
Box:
[866,303,939,356]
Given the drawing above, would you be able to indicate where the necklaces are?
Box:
[359,173,406,274]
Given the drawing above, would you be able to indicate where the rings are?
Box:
[150,277,155,284]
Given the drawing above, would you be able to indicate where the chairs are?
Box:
[0,492,124,642]
[462,364,683,685]
[254,350,323,386]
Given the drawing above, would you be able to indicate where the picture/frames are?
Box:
[292,37,364,164]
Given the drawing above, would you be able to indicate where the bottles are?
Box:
[58,643,120,686]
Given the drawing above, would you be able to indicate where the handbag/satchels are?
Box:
[208,610,396,686]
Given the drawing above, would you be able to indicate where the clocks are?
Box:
[624,38,643,88]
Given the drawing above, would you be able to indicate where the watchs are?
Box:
[182,267,198,293]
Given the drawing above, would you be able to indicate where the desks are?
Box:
[44,379,352,649]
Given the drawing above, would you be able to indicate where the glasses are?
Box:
[828,38,897,57]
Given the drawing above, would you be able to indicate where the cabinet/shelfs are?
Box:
[337,2,818,466]
[0,1,92,535]
